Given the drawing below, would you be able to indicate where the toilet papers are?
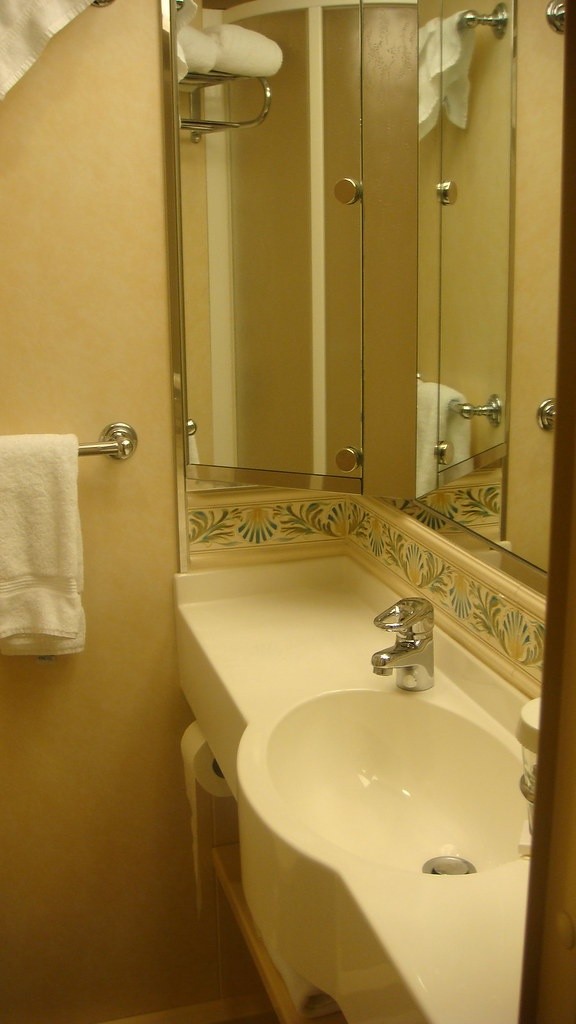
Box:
[180,718,234,799]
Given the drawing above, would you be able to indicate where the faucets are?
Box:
[521,761,537,802]
[371,596,437,692]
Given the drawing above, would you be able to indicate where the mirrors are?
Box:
[160,0,565,598]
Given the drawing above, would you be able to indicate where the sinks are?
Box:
[228,682,529,878]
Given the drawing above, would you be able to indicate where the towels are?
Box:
[0,432,88,657]
[0,0,95,98]
[416,376,472,498]
[174,0,283,93]
[418,8,475,147]
